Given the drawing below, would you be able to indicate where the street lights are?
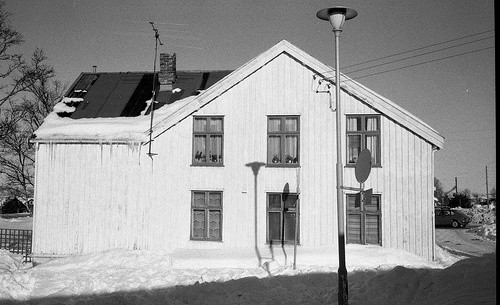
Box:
[316,4,359,305]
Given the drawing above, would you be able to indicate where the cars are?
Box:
[435,209,470,228]
